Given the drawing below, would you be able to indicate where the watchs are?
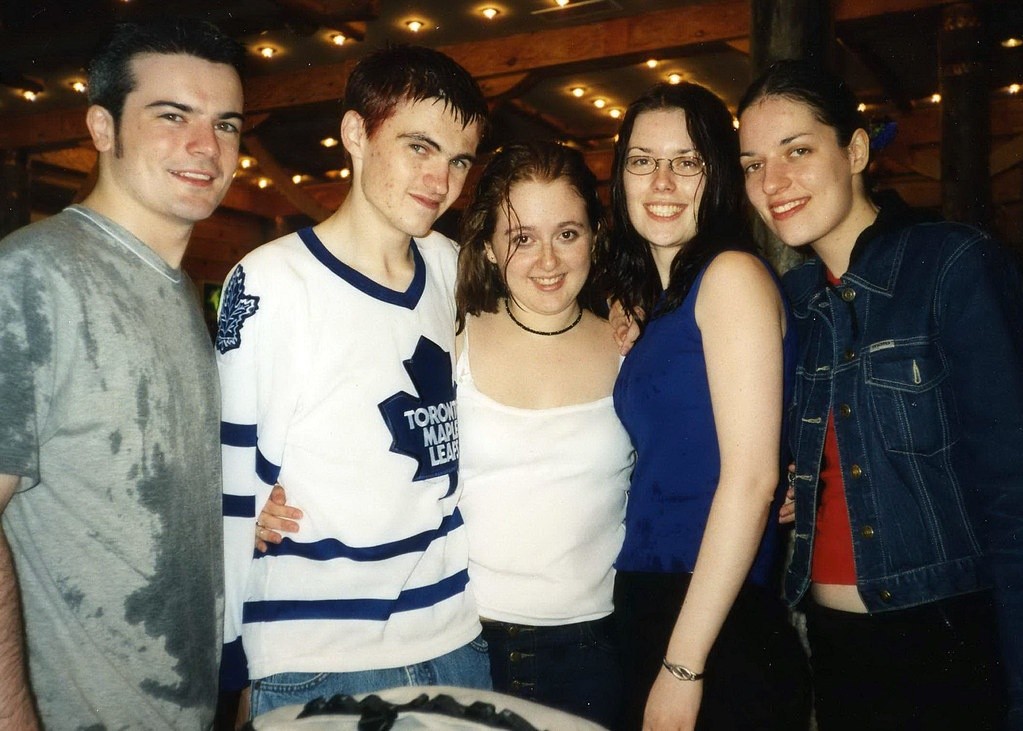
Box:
[662,656,704,681]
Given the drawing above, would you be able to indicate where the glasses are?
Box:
[624,155,710,177]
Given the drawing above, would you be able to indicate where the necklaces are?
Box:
[504,294,583,335]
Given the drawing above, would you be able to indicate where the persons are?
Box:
[0,24,246,731]
[607,59,1023,731]
[252,141,797,731]
[604,83,812,731]
[213,45,646,731]
